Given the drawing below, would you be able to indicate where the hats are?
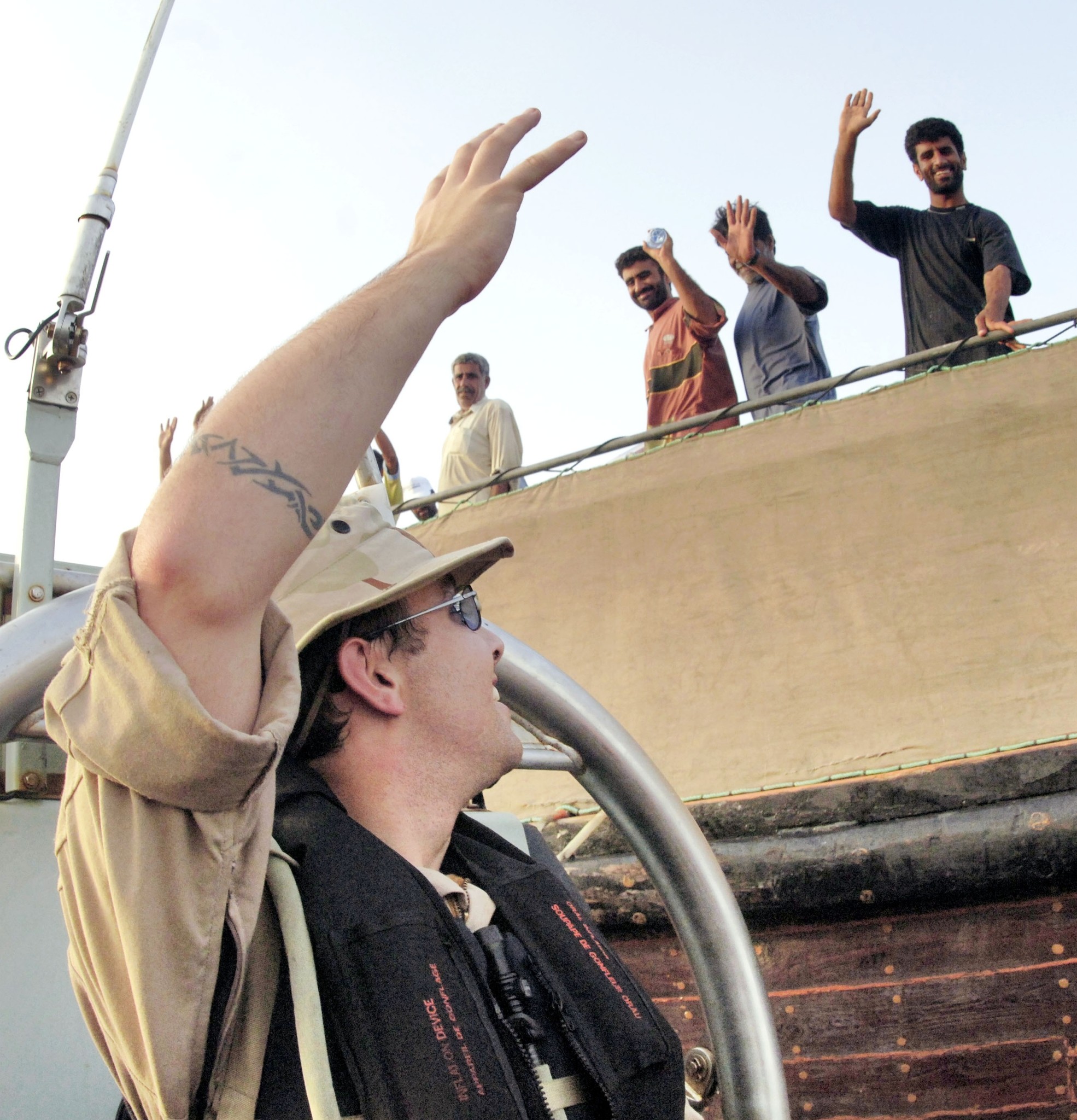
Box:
[271,500,515,648]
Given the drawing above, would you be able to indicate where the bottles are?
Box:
[645,227,668,249]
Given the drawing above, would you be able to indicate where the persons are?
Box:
[435,353,524,518]
[828,88,1031,379]
[372,427,404,526]
[615,228,738,449]
[41,105,687,1120]
[403,475,437,523]
[711,195,829,421]
[158,395,213,483]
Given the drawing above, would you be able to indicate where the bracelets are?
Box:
[742,248,761,267]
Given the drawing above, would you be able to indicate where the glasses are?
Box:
[368,582,482,631]
